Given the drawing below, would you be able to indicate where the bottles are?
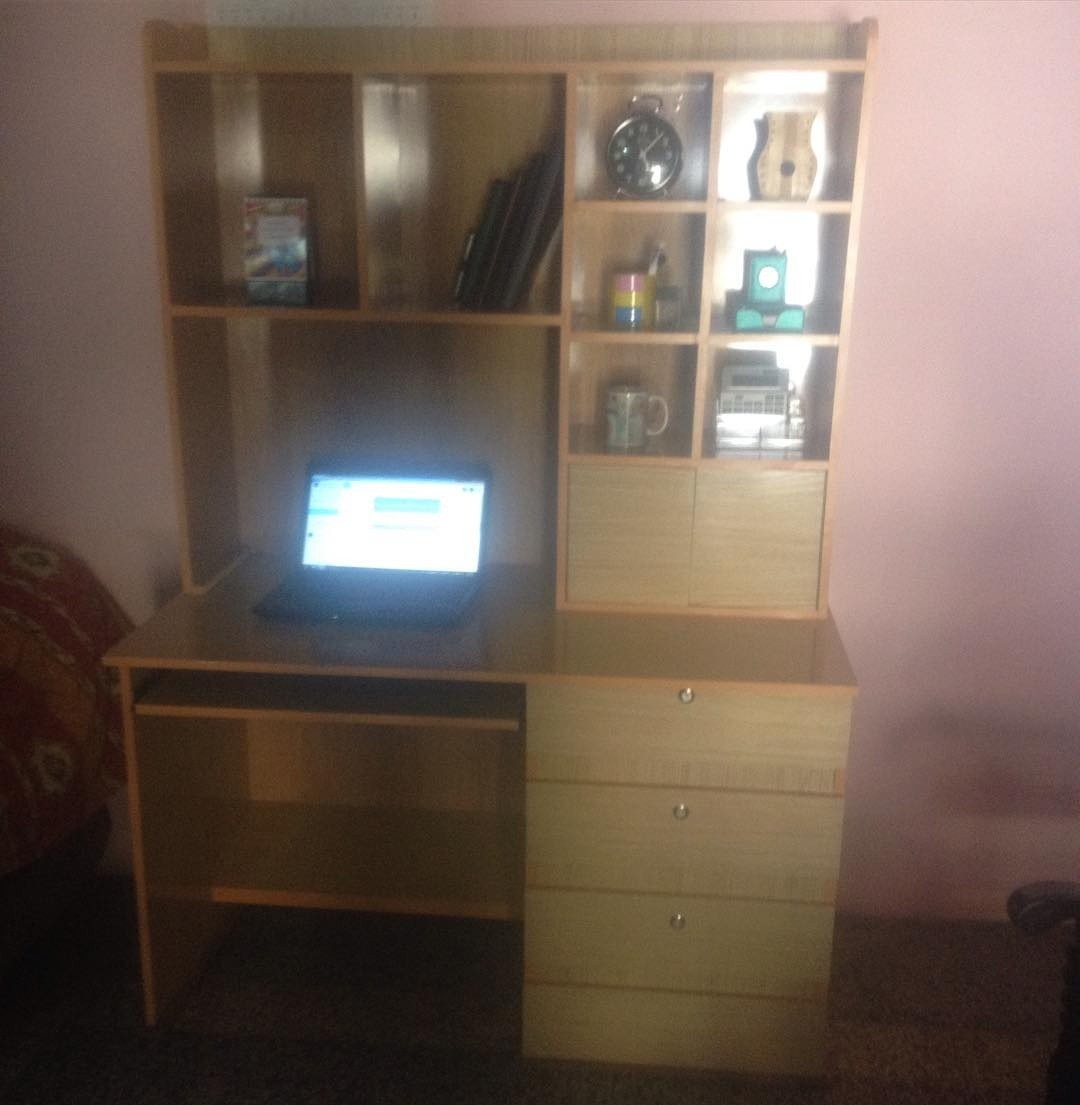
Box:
[755,111,818,202]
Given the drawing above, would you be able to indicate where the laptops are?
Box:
[249,451,494,630]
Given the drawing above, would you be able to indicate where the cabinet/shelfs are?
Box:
[102,21,855,1080]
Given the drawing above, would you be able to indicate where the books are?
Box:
[448,148,564,310]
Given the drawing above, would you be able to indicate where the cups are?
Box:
[603,383,669,451]
[607,269,657,332]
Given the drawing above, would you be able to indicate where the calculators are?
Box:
[717,366,790,423]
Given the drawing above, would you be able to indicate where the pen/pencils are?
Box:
[649,240,663,276]
